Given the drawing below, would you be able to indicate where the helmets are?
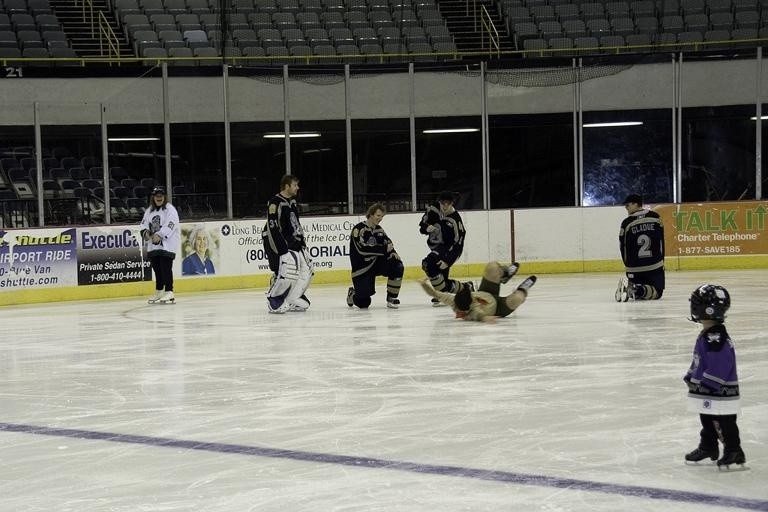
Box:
[151,185,166,196]
[690,284,730,323]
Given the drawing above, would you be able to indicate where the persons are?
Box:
[684,284,745,466]
[182,229,215,275]
[139,186,179,301]
[615,193,666,301]
[419,192,474,305]
[346,203,404,309]
[418,262,537,324]
[268,175,312,313]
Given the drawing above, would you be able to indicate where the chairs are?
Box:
[0,0,83,66]
[0,126,187,227]
[500,0,768,58]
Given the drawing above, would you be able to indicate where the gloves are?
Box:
[142,229,151,241]
[152,233,162,245]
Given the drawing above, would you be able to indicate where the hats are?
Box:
[622,193,642,204]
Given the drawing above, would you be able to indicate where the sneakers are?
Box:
[431,297,442,302]
[387,293,399,304]
[466,280,475,292]
[717,447,745,466]
[347,289,354,306]
[615,278,632,302]
[503,263,519,284]
[149,290,175,301]
[685,442,719,461]
[517,276,536,288]
